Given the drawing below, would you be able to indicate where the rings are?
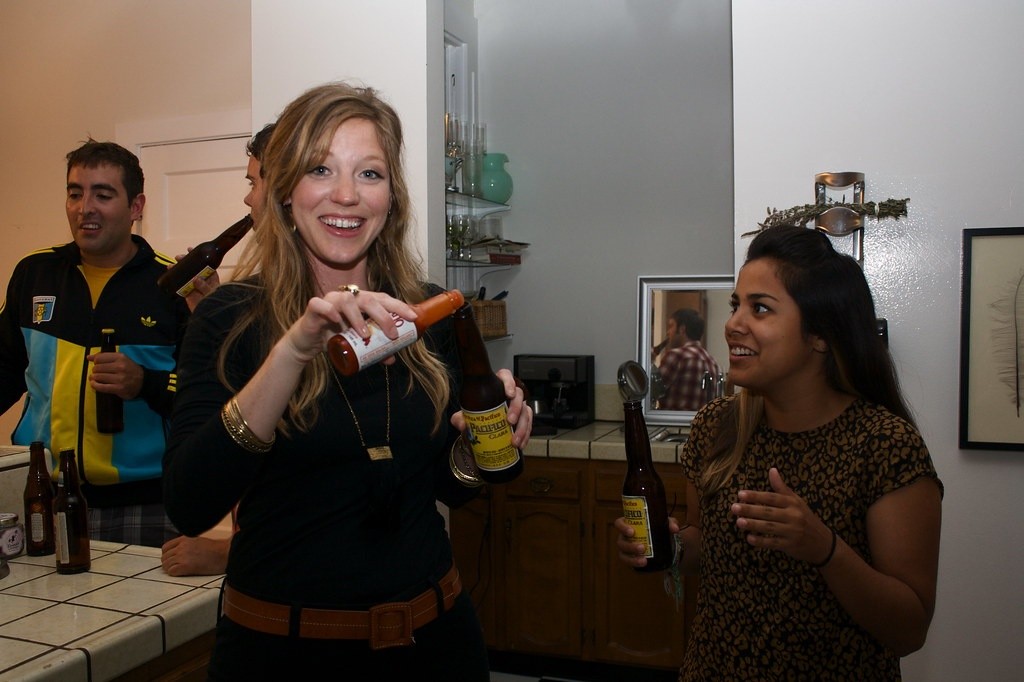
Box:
[338,285,359,294]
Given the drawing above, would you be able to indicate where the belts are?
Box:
[223,556,463,651]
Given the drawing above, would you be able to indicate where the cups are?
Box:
[445,110,488,262]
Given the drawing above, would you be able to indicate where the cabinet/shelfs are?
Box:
[444,154,520,342]
[446,456,697,673]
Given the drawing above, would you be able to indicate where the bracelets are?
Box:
[806,528,836,567]
[450,434,483,487]
[665,533,685,613]
[222,397,275,454]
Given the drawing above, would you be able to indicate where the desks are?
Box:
[0,538,226,682]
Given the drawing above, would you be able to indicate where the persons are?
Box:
[161,124,275,576]
[161,82,534,682]
[616,224,944,682]
[651,307,718,411]
[0,141,183,549]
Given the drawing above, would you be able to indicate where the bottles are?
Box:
[453,304,524,481]
[480,151,513,205]
[620,399,674,571]
[96,328,124,434]
[22,441,55,557]
[327,287,465,375]
[0,513,24,558]
[158,215,254,301]
[54,449,91,574]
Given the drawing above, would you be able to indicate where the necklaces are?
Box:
[323,350,393,459]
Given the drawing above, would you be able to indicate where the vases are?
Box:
[477,153,513,205]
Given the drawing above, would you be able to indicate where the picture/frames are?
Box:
[958,226,1024,452]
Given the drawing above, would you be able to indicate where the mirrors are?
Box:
[635,273,735,428]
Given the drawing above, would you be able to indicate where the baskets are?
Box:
[469,300,507,337]
